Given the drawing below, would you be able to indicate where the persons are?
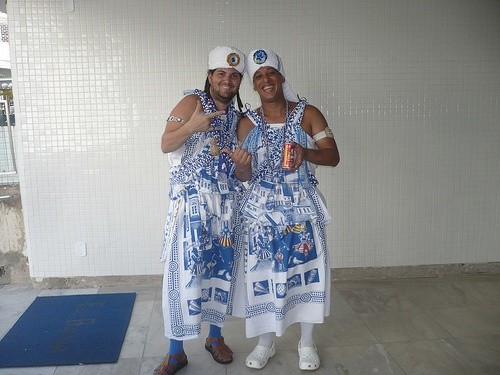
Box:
[223,47,339,371]
[153,44,247,375]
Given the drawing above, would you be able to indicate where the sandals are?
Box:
[245,340,275,369]
[205,335,232,363]
[153,350,188,375]
[298,339,320,371]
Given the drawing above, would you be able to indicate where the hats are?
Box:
[247,49,285,83]
[208,45,246,77]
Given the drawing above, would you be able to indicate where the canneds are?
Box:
[281,143,295,168]
